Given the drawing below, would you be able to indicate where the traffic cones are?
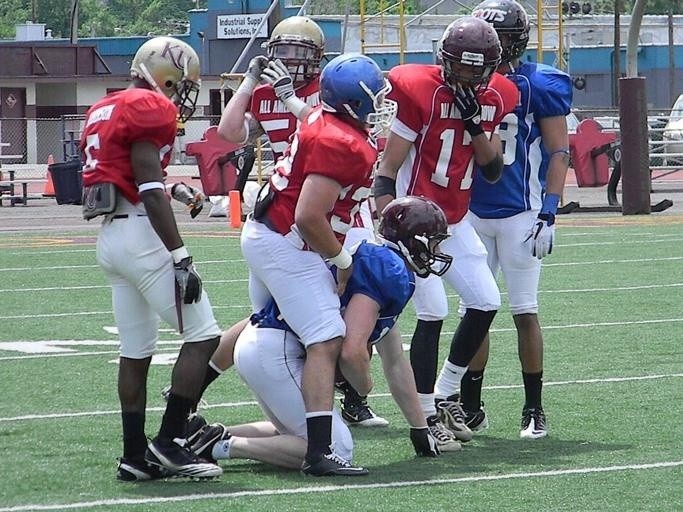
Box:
[41,155,56,198]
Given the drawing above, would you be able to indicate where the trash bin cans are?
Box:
[48,162,80,205]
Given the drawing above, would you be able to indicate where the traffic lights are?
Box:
[560,1,590,14]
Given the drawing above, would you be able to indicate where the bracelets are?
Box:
[169,245,191,265]
[290,97,308,116]
[331,245,354,269]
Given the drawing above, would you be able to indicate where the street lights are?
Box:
[196,29,205,78]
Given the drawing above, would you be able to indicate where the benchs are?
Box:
[0,142,48,207]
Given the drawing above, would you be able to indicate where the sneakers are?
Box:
[340,396,392,428]
[425,394,547,452]
[302,449,369,477]
[116,415,231,484]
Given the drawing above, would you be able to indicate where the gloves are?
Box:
[453,83,486,138]
[261,56,295,103]
[409,427,440,457]
[244,55,268,80]
[522,210,556,259]
[171,181,207,218]
[172,256,202,304]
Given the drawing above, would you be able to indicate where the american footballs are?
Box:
[336,338,372,389]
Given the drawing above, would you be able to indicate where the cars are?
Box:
[165,129,274,178]
[564,93,681,168]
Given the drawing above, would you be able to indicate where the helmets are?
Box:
[319,52,398,150]
[379,195,453,278]
[130,34,200,123]
[436,17,502,94]
[262,17,326,92]
[470,0,530,62]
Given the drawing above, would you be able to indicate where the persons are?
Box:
[216,15,391,426]
[371,16,520,454]
[180,195,455,471]
[459,0,574,439]
[76,36,222,482]
[161,52,385,475]
